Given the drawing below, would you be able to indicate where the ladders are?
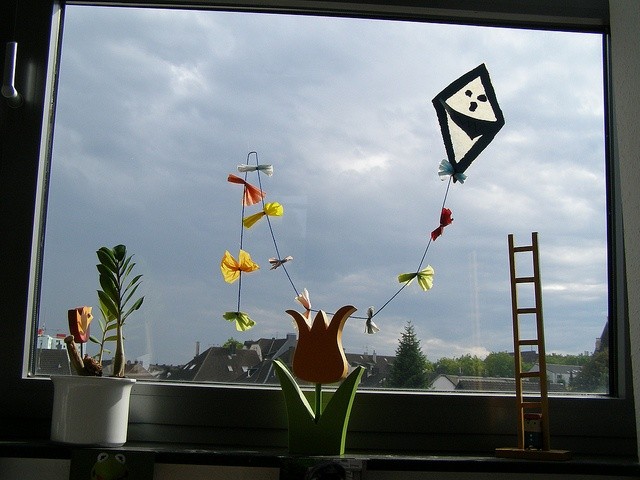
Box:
[508,232,550,452]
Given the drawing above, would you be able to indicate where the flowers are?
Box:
[273,305,364,455]
[65,245,144,377]
